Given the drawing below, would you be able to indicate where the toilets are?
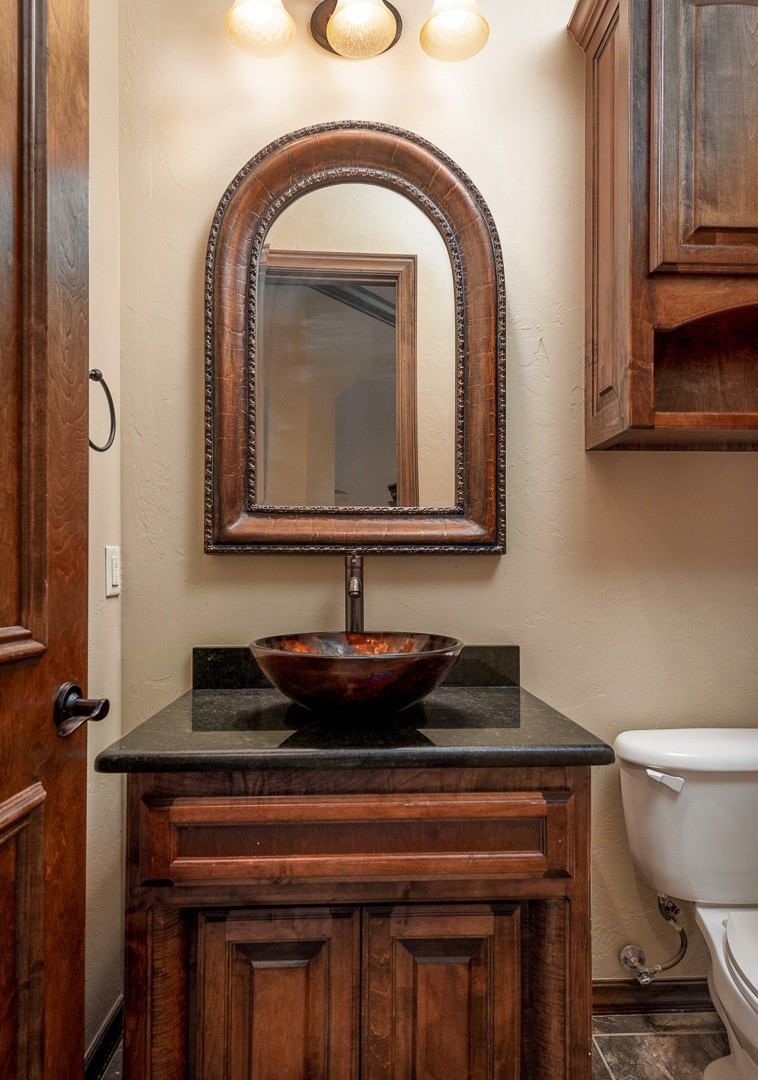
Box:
[612,727,757,1080]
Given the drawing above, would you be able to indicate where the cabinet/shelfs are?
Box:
[123,765,592,1079]
[568,0,758,452]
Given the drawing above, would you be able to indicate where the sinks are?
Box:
[247,631,464,720]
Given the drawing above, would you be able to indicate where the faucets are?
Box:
[343,554,366,634]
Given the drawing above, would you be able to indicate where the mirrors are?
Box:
[203,121,506,556]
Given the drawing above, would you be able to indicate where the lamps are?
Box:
[224,0,489,62]
